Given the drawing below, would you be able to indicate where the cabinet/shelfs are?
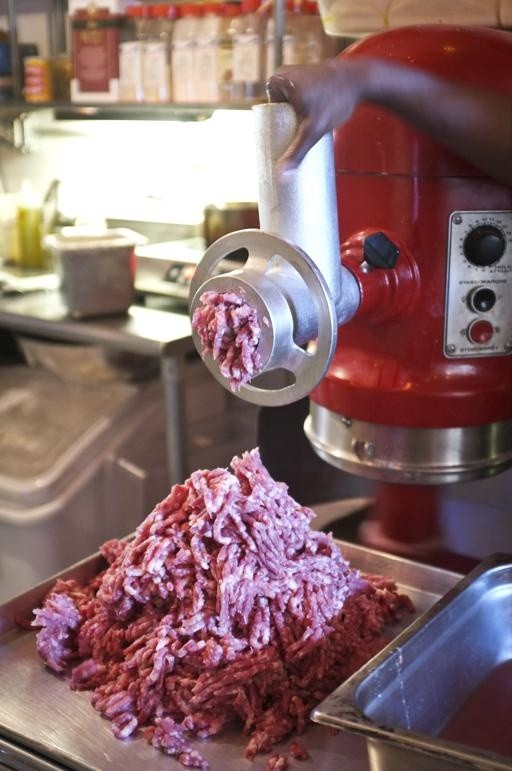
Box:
[0,0,320,130]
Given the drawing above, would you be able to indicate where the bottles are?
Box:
[22,42,52,106]
[117,1,327,103]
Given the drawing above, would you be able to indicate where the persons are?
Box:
[263,53,511,187]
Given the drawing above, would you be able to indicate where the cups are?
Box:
[15,205,46,271]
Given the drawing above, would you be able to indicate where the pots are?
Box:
[204,201,259,258]
